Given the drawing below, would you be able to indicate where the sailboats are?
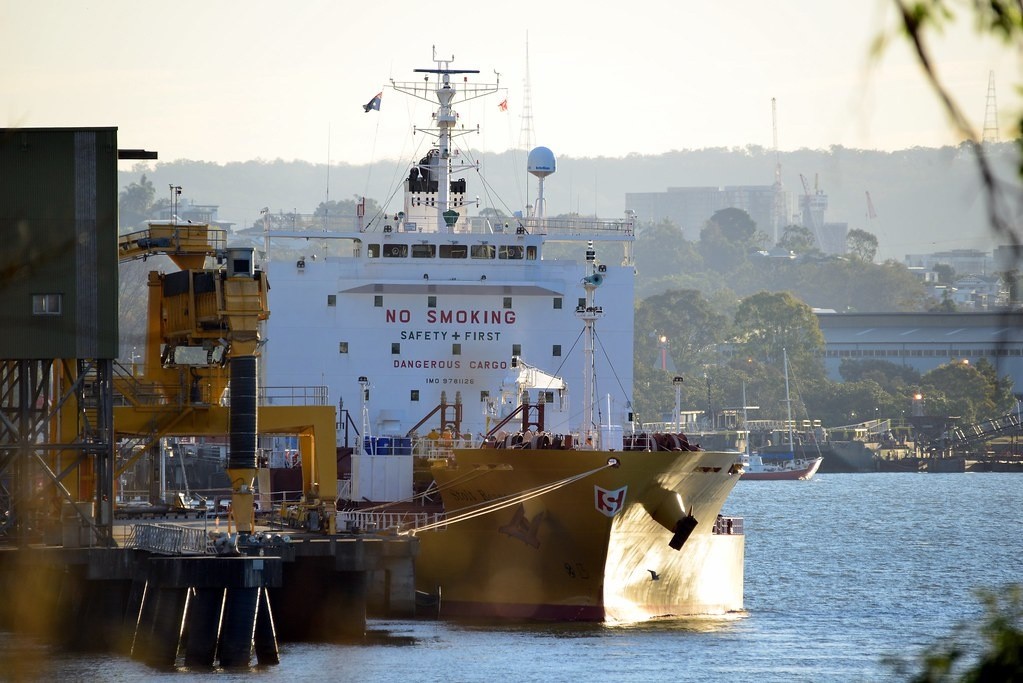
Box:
[739,347,825,481]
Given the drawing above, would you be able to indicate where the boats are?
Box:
[253,24,749,631]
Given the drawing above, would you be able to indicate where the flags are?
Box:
[499,100,507,109]
[362,91,383,113]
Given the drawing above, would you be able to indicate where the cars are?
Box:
[125,495,302,521]
